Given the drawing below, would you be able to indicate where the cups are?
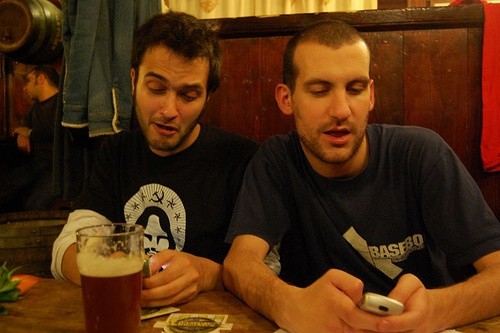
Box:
[76,223,143,333]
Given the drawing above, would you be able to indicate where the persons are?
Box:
[222,19,500,333]
[8,64,63,173]
[48,11,285,309]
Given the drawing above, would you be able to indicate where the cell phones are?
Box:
[141,258,150,278]
[358,292,405,316]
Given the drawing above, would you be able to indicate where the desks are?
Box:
[0,277,500,333]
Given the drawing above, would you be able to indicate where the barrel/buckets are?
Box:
[0,0,66,66]
